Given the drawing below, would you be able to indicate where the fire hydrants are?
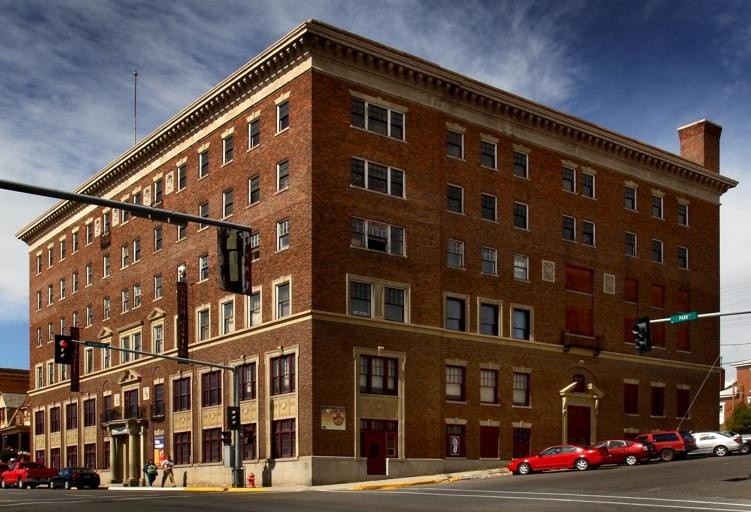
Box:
[246,472,255,488]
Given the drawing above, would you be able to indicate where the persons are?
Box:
[143,459,158,486]
[161,455,176,487]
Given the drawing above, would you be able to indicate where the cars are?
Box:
[0,463,11,476]
[718,431,751,455]
[593,440,656,468]
[506,444,609,476]
[687,431,742,458]
[46,467,100,491]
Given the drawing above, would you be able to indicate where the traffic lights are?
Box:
[631,315,651,353]
[54,334,72,365]
[220,431,231,446]
[226,406,240,430]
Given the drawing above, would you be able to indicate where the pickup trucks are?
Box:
[1,461,57,490]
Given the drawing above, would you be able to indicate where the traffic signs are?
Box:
[84,341,107,348]
[671,310,698,324]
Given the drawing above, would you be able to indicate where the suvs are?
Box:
[633,430,698,462]
